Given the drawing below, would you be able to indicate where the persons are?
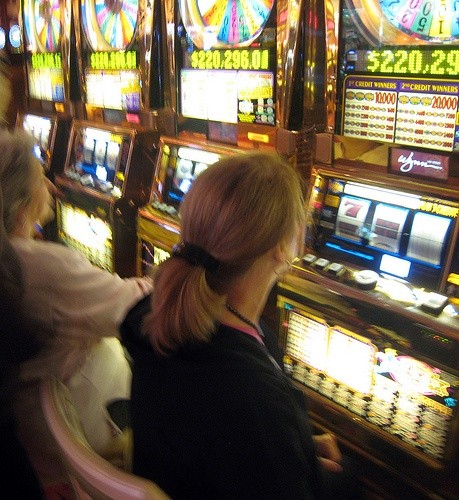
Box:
[0,126,155,379]
[114,150,348,500]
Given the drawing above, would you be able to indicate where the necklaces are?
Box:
[222,301,261,333]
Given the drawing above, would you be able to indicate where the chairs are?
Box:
[38,374,173,500]
